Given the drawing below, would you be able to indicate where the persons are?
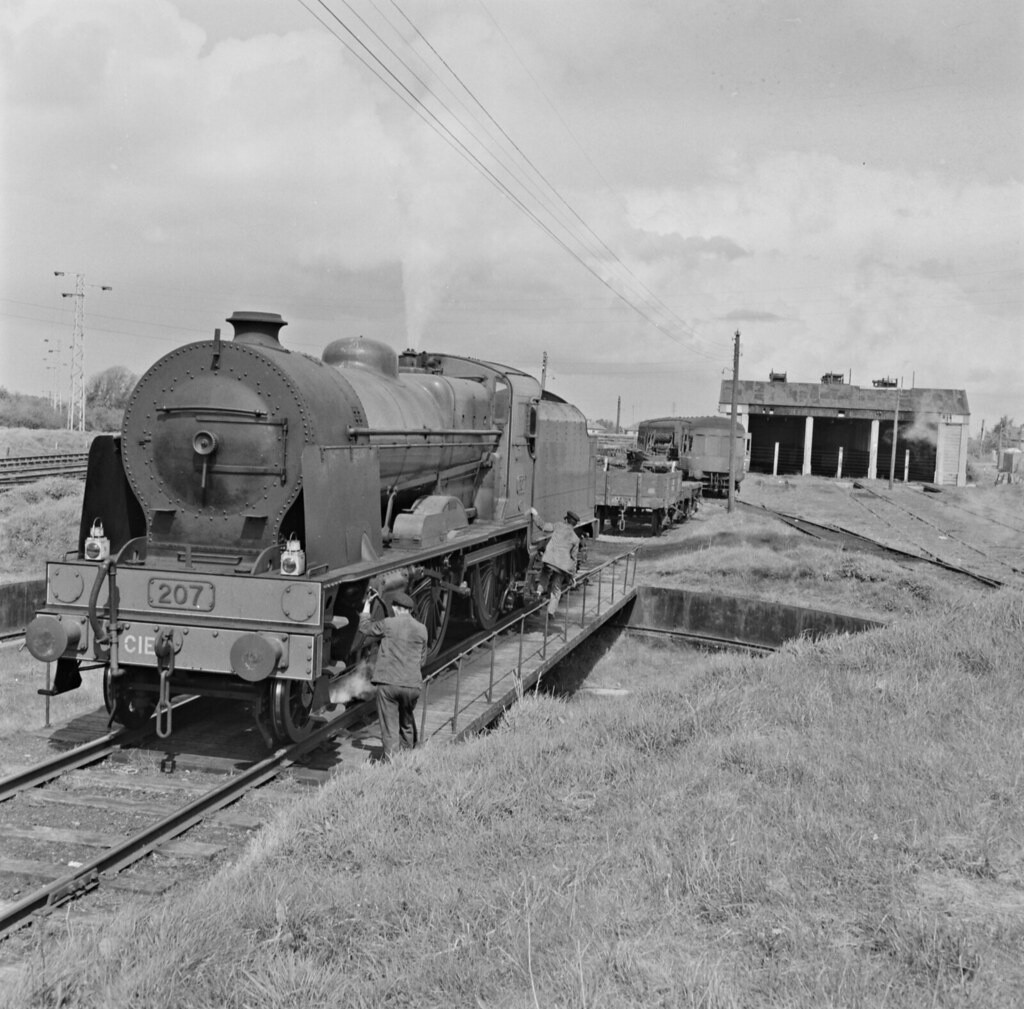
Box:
[530,507,580,621]
[358,591,427,762]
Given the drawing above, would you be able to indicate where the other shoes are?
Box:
[547,613,555,622]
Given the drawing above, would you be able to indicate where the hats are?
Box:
[392,591,414,607]
[564,511,581,521]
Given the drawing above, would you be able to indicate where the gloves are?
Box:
[531,507,537,515]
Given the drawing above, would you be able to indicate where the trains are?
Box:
[24,310,704,738]
[627,416,749,500]
